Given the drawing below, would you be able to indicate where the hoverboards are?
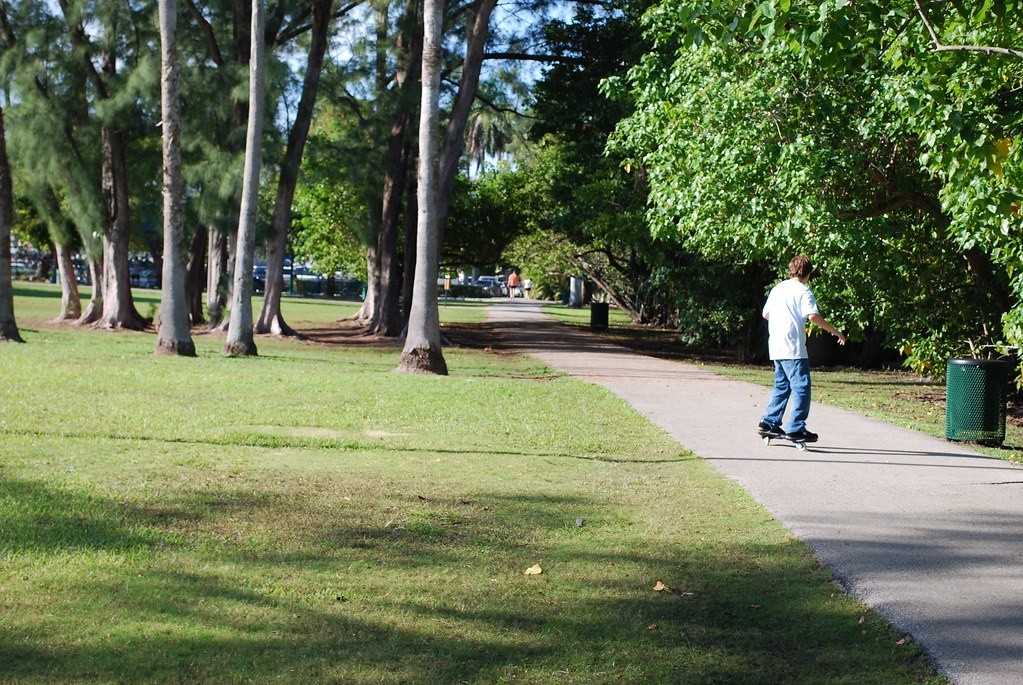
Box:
[758,429,806,451]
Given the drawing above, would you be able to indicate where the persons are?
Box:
[507,269,519,300]
[757,255,845,442]
[523,277,533,301]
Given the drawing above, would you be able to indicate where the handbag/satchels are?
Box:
[514,287,521,296]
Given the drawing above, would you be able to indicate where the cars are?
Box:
[10,250,523,297]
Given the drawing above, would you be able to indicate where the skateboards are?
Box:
[757,429,817,452]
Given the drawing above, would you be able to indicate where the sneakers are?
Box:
[785,426,818,441]
[757,419,785,434]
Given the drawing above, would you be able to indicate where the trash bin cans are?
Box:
[590,303,609,328]
[568,275,584,308]
[945,359,1009,447]
